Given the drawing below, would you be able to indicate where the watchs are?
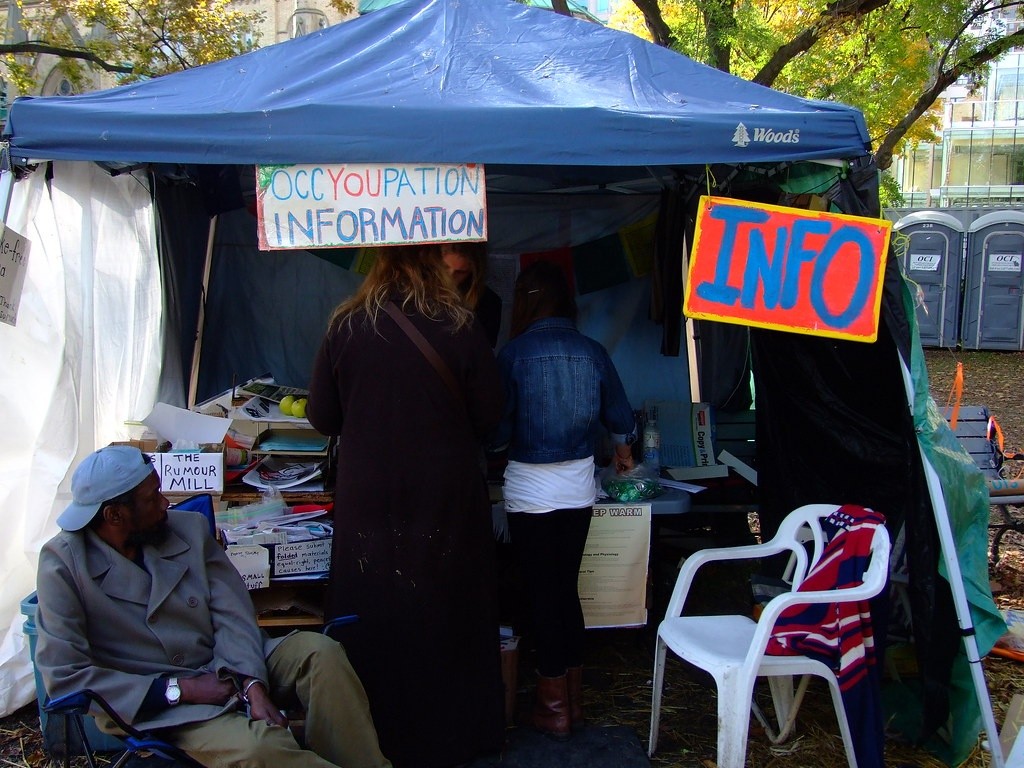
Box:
[165,678,181,706]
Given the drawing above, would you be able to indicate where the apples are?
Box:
[279,396,308,417]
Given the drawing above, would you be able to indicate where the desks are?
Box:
[577,469,692,681]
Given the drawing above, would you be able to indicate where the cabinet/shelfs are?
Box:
[218,468,333,627]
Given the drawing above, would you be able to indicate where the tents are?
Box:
[1,0,1008,767]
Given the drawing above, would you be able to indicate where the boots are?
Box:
[568,664,585,732]
[514,665,571,742]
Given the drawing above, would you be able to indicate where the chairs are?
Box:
[647,504,892,768]
[40,491,360,768]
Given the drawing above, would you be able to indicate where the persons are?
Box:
[304,244,504,767]
[441,241,503,348]
[35,445,393,768]
[494,260,637,742]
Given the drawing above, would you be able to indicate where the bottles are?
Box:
[642,419,661,477]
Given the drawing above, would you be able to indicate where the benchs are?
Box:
[715,405,1024,562]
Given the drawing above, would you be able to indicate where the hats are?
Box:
[56,445,154,532]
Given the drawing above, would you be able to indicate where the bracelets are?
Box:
[242,679,266,701]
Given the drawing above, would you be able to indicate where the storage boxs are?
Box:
[499,646,519,721]
[108,440,226,495]
[219,519,334,578]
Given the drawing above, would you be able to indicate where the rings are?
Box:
[267,719,273,725]
